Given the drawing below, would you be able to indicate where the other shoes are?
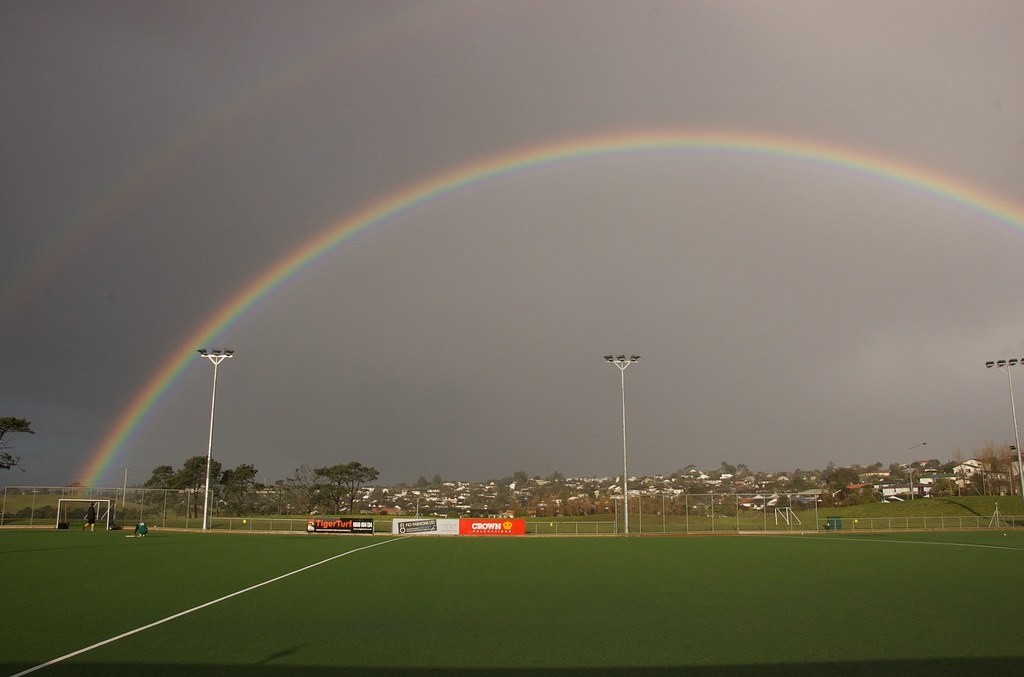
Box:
[91,529,96,532]
[81,526,84,530]
[139,534,146,537]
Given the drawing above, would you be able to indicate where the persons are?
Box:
[82,503,96,532]
[134,520,148,537]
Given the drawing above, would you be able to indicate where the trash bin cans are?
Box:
[827,516,842,530]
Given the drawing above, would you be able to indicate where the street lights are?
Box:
[986,358,1024,496]
[604,353,641,533]
[197,348,236,531]
